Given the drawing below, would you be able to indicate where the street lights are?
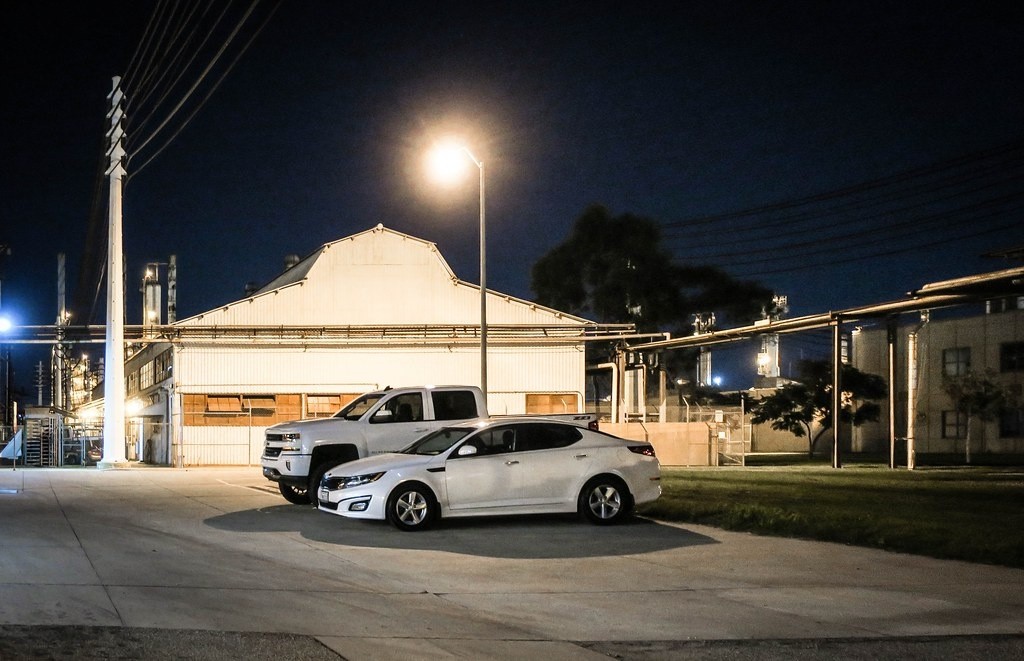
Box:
[428,142,488,406]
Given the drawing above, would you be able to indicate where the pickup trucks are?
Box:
[27,427,104,464]
[259,385,600,507]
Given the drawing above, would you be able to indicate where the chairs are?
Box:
[394,403,412,421]
[491,430,513,452]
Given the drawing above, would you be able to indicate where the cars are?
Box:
[316,416,664,533]
